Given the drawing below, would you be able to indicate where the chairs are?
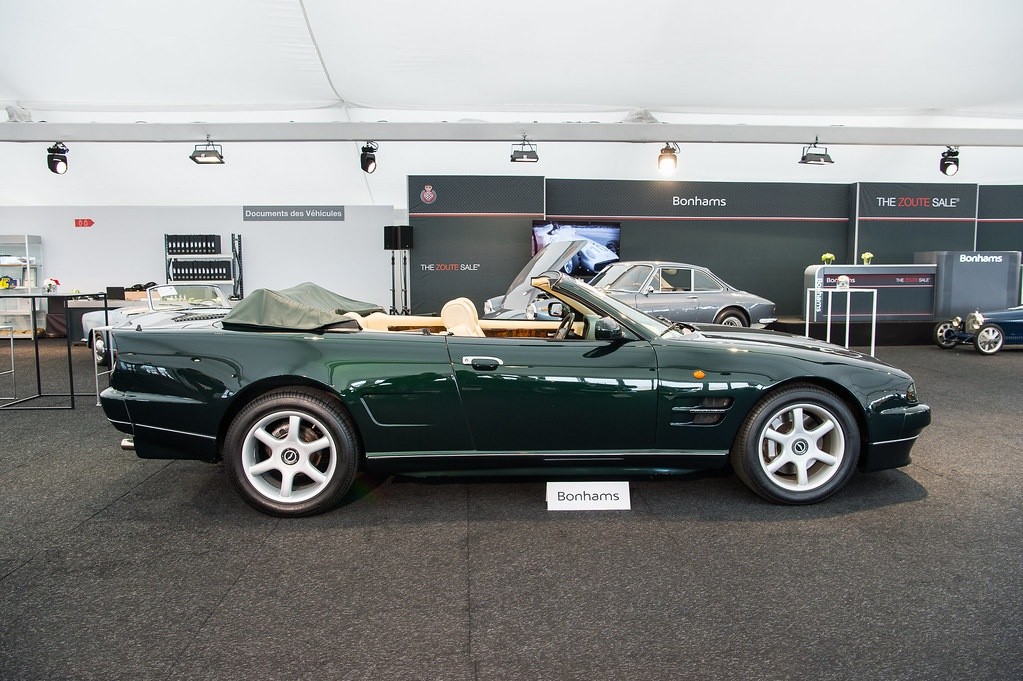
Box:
[331,294,488,338]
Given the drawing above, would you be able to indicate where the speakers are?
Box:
[383,225,414,251]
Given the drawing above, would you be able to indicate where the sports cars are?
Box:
[91,264,932,516]
[84,280,231,367]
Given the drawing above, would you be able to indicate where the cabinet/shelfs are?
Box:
[0,234,46,340]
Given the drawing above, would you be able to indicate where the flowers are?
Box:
[821,252,835,262]
[861,251,873,260]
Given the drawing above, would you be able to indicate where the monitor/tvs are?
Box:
[530,220,622,279]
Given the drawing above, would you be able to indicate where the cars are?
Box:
[482,238,776,334]
[936,300,1023,356]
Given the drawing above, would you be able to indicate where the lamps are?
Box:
[189,143,226,166]
[359,145,376,174]
[656,146,678,171]
[939,146,961,176]
[510,143,540,163]
[46,146,69,174]
[798,146,837,167]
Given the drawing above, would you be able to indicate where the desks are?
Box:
[1,287,111,415]
[804,284,878,361]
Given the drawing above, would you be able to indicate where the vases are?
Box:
[824,258,831,264]
[864,257,872,265]
